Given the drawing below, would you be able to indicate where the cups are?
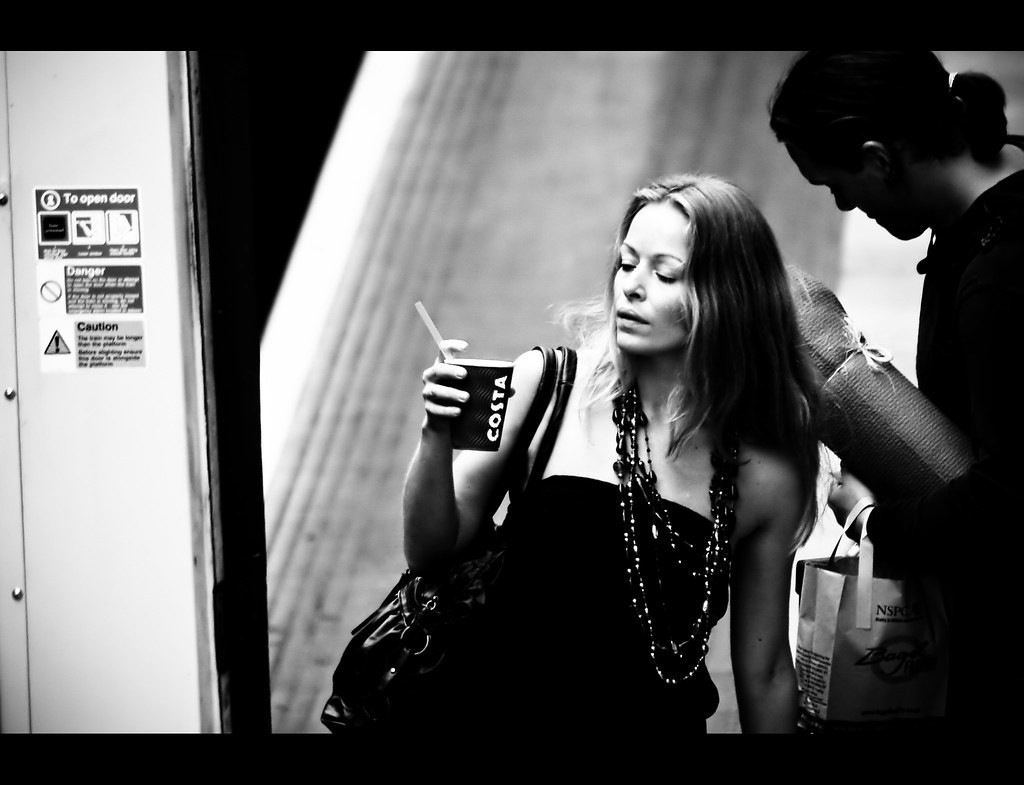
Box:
[443,359,514,451]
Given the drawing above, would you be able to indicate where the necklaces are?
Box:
[612,384,738,684]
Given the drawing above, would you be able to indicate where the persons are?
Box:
[766,45,1024,728]
[404,177,816,731]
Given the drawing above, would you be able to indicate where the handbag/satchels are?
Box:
[320,345,578,735]
[792,495,926,735]
